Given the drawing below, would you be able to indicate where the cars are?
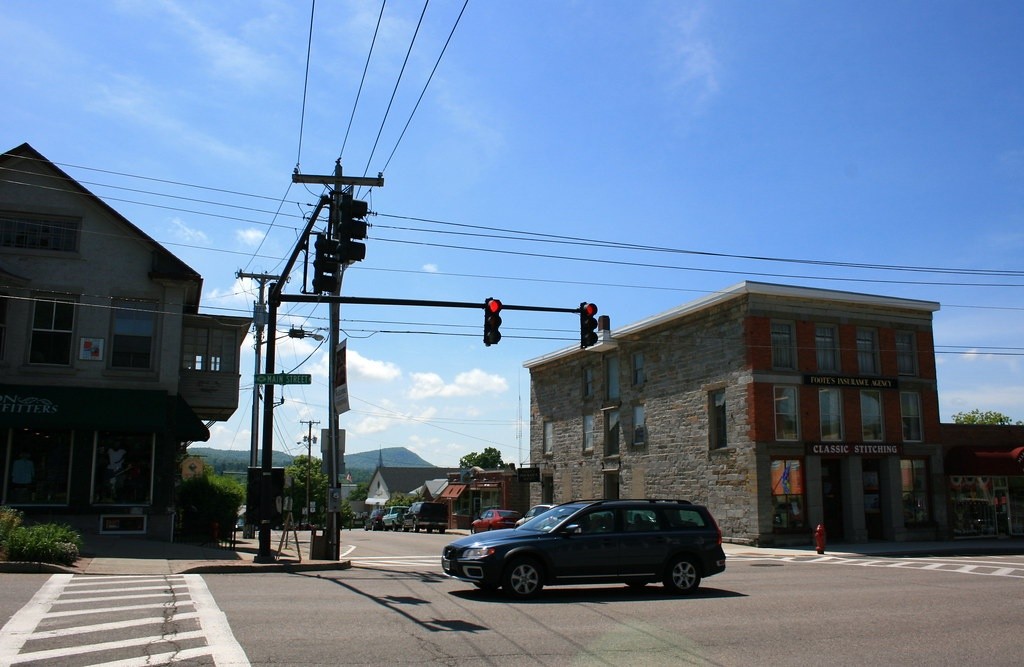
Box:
[471,509,524,534]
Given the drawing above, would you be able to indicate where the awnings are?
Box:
[441,484,467,500]
[0,384,210,441]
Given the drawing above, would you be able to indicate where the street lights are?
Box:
[295,441,311,524]
[243,332,326,539]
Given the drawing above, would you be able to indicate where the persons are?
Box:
[104,442,127,480]
[375,513,382,531]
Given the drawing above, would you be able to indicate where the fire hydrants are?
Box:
[814,523,828,555]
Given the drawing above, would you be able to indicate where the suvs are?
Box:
[363,505,410,532]
[400,501,449,534]
[440,498,728,599]
[513,504,563,529]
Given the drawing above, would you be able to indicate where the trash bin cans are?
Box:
[309,526,326,559]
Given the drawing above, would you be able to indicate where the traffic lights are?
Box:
[334,194,369,265]
[577,300,600,348]
[482,297,504,346]
